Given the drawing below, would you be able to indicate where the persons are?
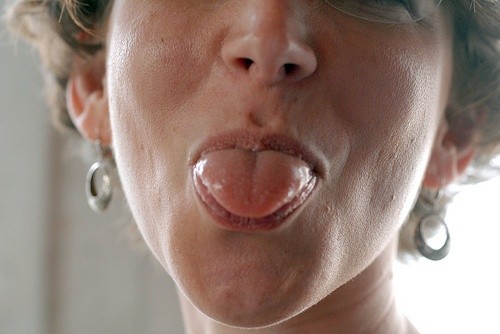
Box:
[1,1,499,334]
